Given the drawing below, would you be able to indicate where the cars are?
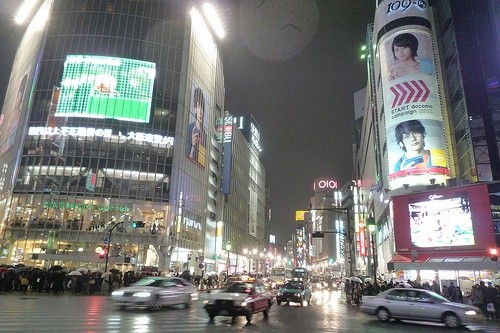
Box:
[263,267,338,292]
[358,287,485,328]
[276,280,312,307]
[202,280,276,322]
[110,276,194,313]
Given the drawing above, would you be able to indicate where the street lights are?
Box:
[243,249,288,278]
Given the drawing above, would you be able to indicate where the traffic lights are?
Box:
[366,217,377,235]
[490,248,498,261]
[225,240,231,251]
[132,223,145,227]
[99,250,104,259]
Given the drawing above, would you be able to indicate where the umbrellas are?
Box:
[345,277,371,283]
[68,268,120,278]
[49,265,63,271]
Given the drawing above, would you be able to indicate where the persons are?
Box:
[185,87,207,160]
[180,270,219,289]
[394,120,432,171]
[5,74,27,149]
[377,279,463,303]
[0,269,161,295]
[469,281,500,319]
[390,33,420,80]
[345,279,375,305]
